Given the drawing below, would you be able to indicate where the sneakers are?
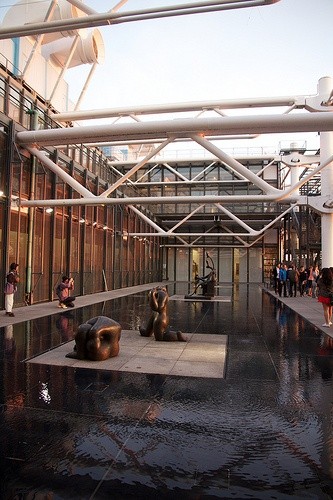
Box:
[56,301,61,308]
[322,323,330,328]
[329,321,332,325]
[60,303,67,309]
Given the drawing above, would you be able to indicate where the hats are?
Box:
[10,263,19,268]
[62,276,68,282]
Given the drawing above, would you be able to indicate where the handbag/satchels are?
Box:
[318,295,330,305]
[4,283,13,294]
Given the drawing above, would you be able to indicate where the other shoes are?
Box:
[6,312,15,317]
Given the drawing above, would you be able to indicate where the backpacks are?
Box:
[56,282,65,297]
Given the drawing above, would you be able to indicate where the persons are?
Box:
[316,267,333,328]
[4,262,19,317]
[56,277,76,308]
[273,262,319,299]
